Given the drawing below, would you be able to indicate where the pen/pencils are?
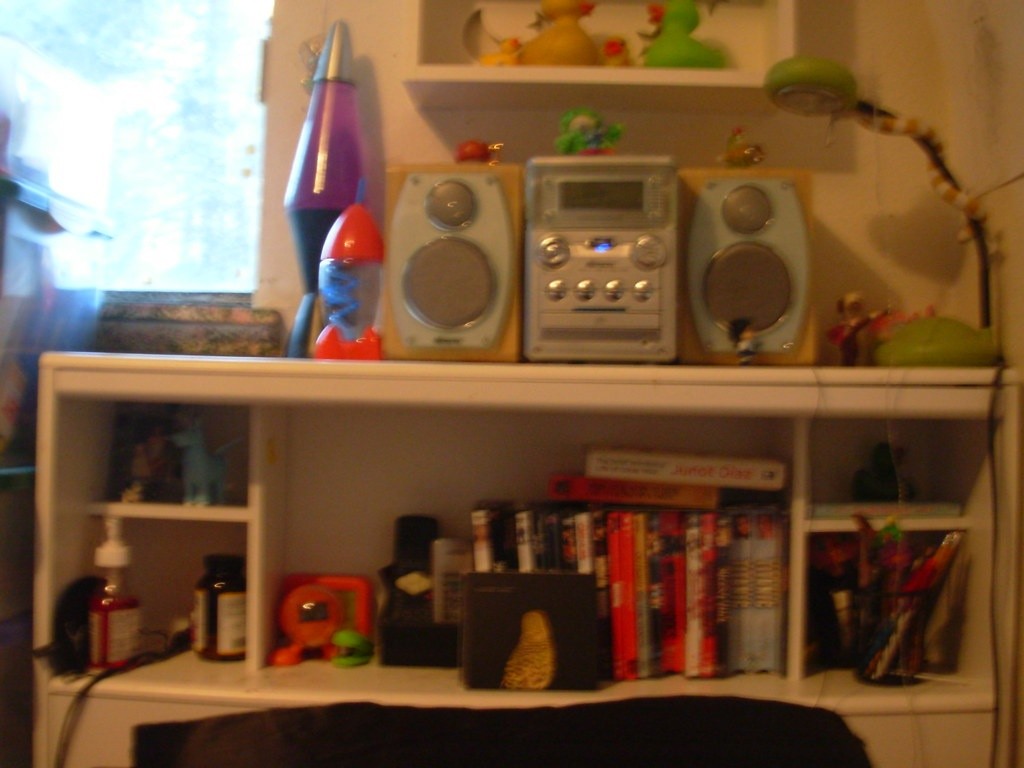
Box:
[859,528,969,684]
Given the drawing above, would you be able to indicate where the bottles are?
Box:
[193,551,247,662]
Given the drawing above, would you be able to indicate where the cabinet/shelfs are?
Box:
[31,349,1021,768]
[397,0,797,115]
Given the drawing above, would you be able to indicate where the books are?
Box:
[584,450,786,491]
[544,473,718,509]
[459,569,602,692]
[469,498,786,681]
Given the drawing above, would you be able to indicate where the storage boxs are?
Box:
[459,573,603,692]
[377,596,460,669]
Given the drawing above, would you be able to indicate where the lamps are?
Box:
[768,55,1003,367]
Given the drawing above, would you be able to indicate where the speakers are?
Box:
[377,165,522,361]
[674,166,820,365]
